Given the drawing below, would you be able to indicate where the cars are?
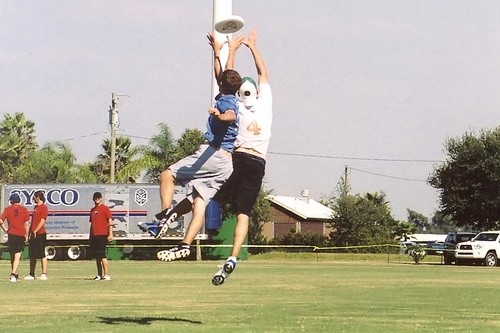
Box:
[425,243,447,254]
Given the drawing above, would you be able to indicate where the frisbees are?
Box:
[214,15,245,34]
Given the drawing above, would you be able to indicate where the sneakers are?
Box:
[137,221,160,238]
[212,260,237,286]
[100,276,111,280]
[24,275,35,280]
[39,274,47,280]
[10,273,20,282]
[94,275,102,280]
[156,242,190,261]
[155,209,177,238]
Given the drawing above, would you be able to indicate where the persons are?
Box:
[137,28,273,286]
[89,191,113,280]
[24,191,48,279]
[1,196,30,283]
[155,35,246,262]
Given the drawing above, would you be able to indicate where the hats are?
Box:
[239,77,257,106]
[93,192,102,198]
[9,194,19,201]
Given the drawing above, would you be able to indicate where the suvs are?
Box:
[444,233,477,264]
[454,230,500,265]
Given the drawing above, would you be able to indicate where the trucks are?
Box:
[0,183,222,260]
